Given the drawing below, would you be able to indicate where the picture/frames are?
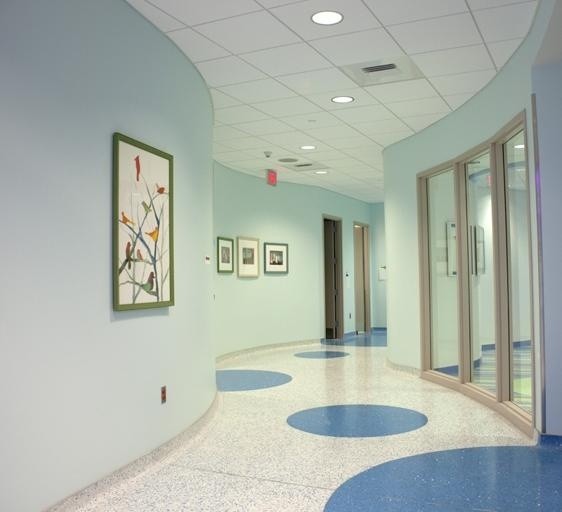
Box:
[237,235,259,278]
[112,132,174,313]
[216,236,234,274]
[264,241,289,275]
